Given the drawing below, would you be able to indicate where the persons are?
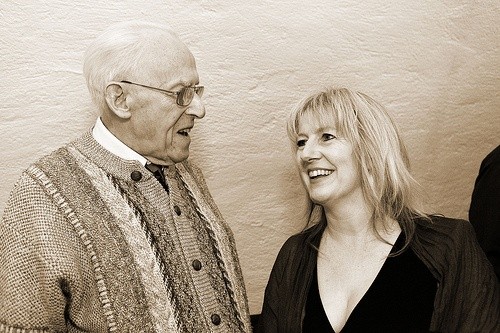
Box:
[0,21,252,333]
[261,88,500,333]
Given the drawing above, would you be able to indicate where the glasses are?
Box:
[120,80,204,107]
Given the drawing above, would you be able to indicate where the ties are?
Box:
[146,163,169,193]
